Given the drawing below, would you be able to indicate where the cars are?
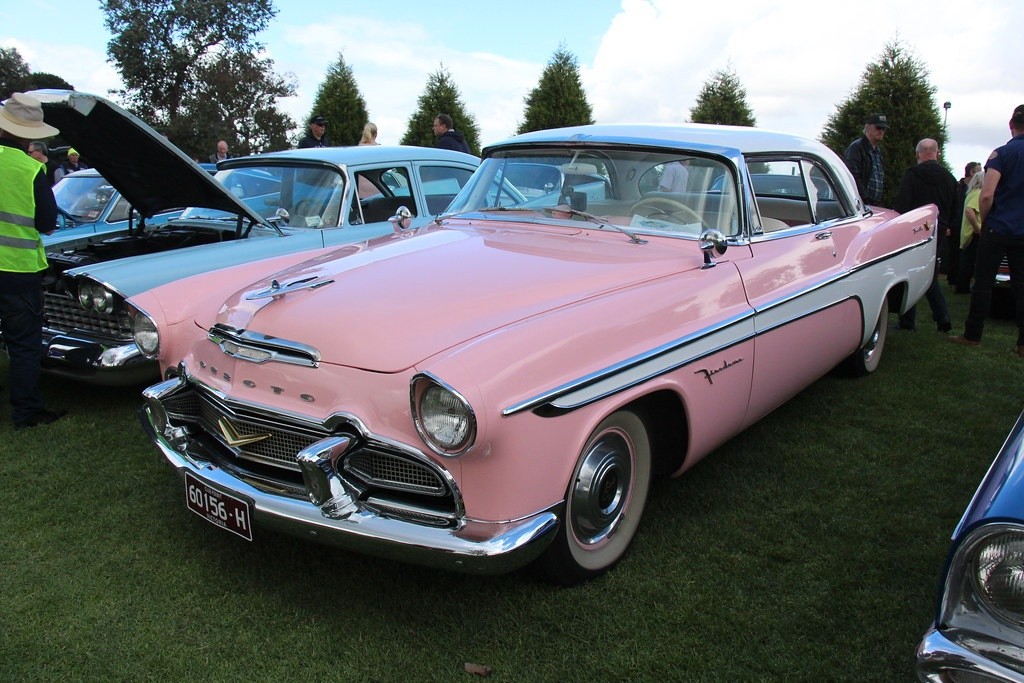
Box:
[50,163,341,226]
[915,407,1024,683]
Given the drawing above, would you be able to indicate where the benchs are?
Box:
[641,191,840,229]
[362,195,455,224]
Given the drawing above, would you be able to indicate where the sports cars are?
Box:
[141,121,939,581]
[28,90,614,395]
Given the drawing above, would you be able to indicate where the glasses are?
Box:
[27,150,36,154]
[319,123,325,127]
[877,127,884,130]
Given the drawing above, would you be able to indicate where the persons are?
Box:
[888,139,957,331]
[947,163,985,294]
[359,122,381,200]
[640,159,694,193]
[0,92,67,428]
[844,115,890,206]
[208,141,231,164]
[433,114,470,153]
[29,142,64,186]
[950,104,1024,357]
[61,148,88,175]
[297,116,334,188]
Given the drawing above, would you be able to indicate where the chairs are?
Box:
[587,202,668,222]
[666,211,790,231]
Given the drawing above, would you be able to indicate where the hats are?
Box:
[865,116,890,133]
[312,116,328,124]
[68,148,80,157]
[438,114,454,129]
[0,92,60,139]
[1012,105,1024,123]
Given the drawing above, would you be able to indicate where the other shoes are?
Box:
[949,335,981,347]
[890,321,916,331]
[937,322,952,332]
[14,409,60,430]
[1015,346,1024,356]
